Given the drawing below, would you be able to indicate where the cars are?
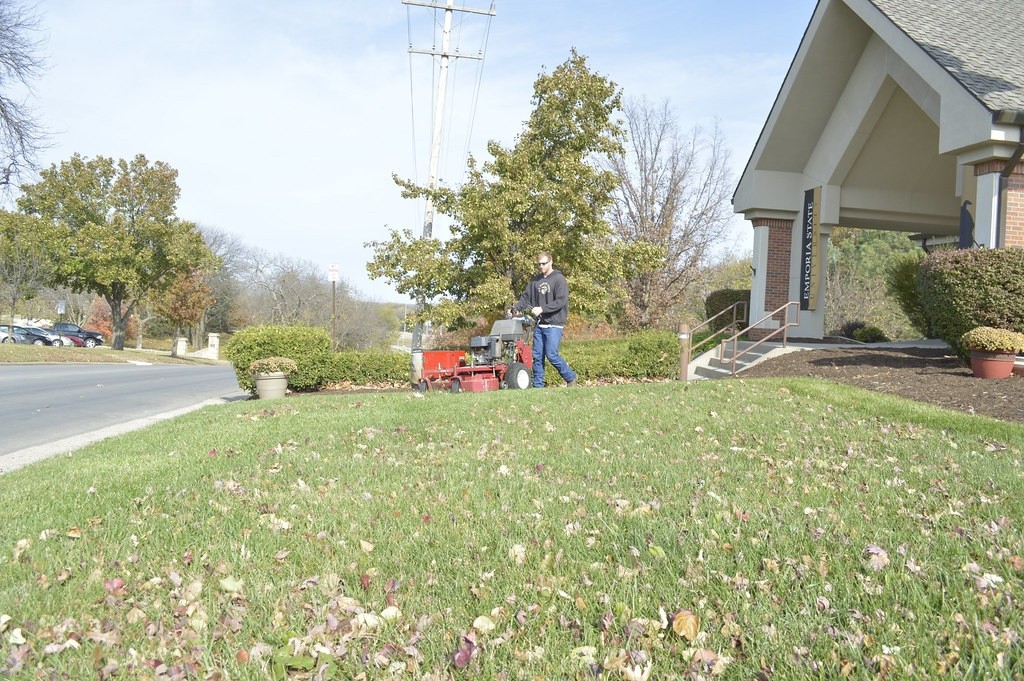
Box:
[0,325,85,352]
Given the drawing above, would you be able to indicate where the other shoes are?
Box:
[567,373,578,387]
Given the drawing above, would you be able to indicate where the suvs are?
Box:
[54,323,104,349]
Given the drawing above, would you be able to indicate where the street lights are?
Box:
[328,263,338,351]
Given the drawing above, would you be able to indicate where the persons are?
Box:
[507,252,579,388]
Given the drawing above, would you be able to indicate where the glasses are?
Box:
[538,260,551,266]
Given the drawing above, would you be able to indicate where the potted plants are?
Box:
[959,326,1024,380]
[250,356,299,399]
